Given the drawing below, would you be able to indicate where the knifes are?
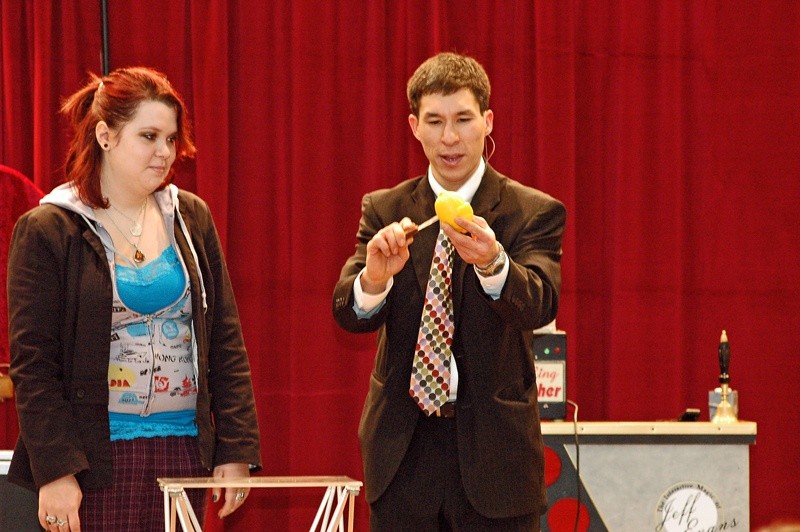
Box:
[404,213,439,240]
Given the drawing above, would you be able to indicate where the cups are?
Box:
[708,390,738,421]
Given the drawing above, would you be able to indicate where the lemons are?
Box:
[435,191,474,234]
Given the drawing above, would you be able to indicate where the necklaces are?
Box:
[100,197,148,263]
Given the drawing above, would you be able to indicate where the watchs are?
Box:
[475,240,506,276]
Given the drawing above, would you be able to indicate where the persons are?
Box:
[332,54,567,532]
[0,66,263,532]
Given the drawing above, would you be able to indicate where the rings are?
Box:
[46,515,57,525]
[57,518,68,526]
[236,491,245,502]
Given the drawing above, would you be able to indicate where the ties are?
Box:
[409,218,454,416]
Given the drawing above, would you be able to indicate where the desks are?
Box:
[539,420,757,532]
[156,475,363,532]
